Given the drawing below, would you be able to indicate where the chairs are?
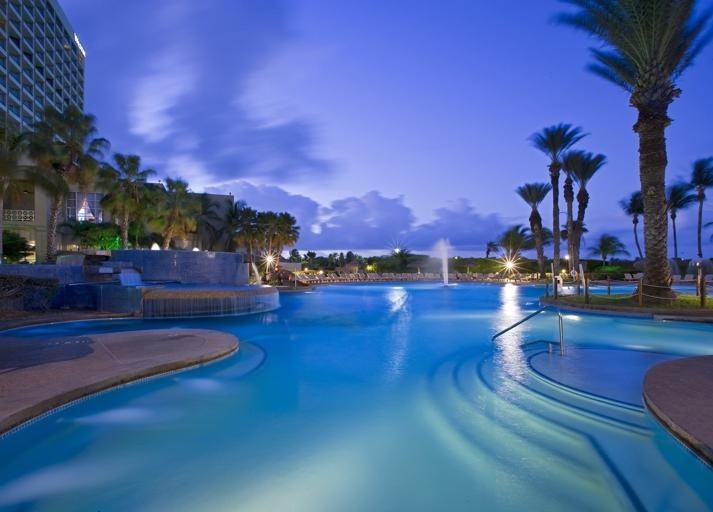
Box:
[624,273,713,285]
[296,273,540,287]
